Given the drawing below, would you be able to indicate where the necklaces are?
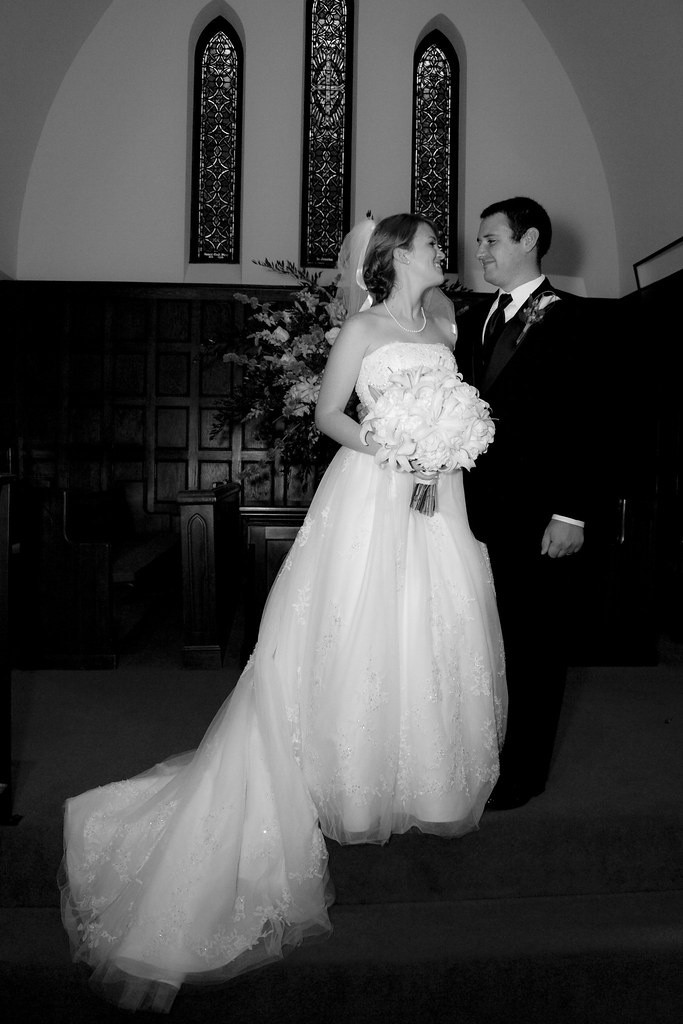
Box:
[383,299,426,332]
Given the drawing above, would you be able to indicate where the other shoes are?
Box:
[485,774,546,811]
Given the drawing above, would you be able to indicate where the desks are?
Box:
[240,501,310,664]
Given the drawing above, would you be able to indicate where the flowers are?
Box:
[516,290,562,345]
[225,249,357,470]
[363,368,495,517]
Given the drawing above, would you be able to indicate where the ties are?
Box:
[484,293,513,348]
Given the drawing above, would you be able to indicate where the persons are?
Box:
[61,213,509,1009]
[461,196,615,810]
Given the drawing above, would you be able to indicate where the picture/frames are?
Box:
[68,296,317,508]
[633,235,683,291]
